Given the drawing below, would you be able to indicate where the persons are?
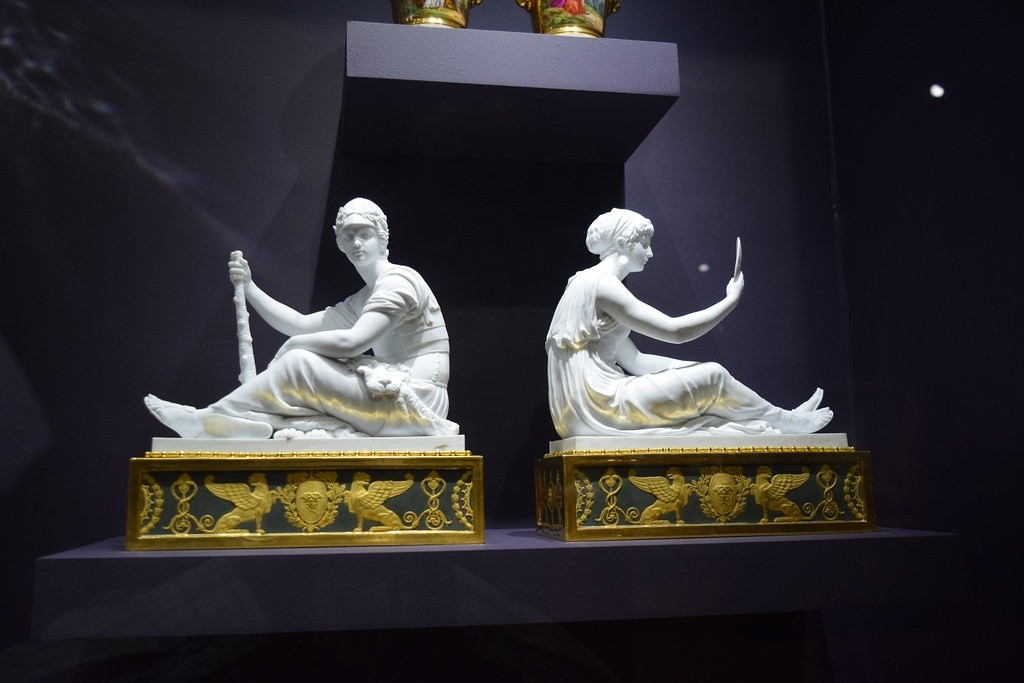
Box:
[546,208,833,439]
[142,197,450,438]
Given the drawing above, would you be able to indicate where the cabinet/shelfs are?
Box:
[35,21,972,640]
